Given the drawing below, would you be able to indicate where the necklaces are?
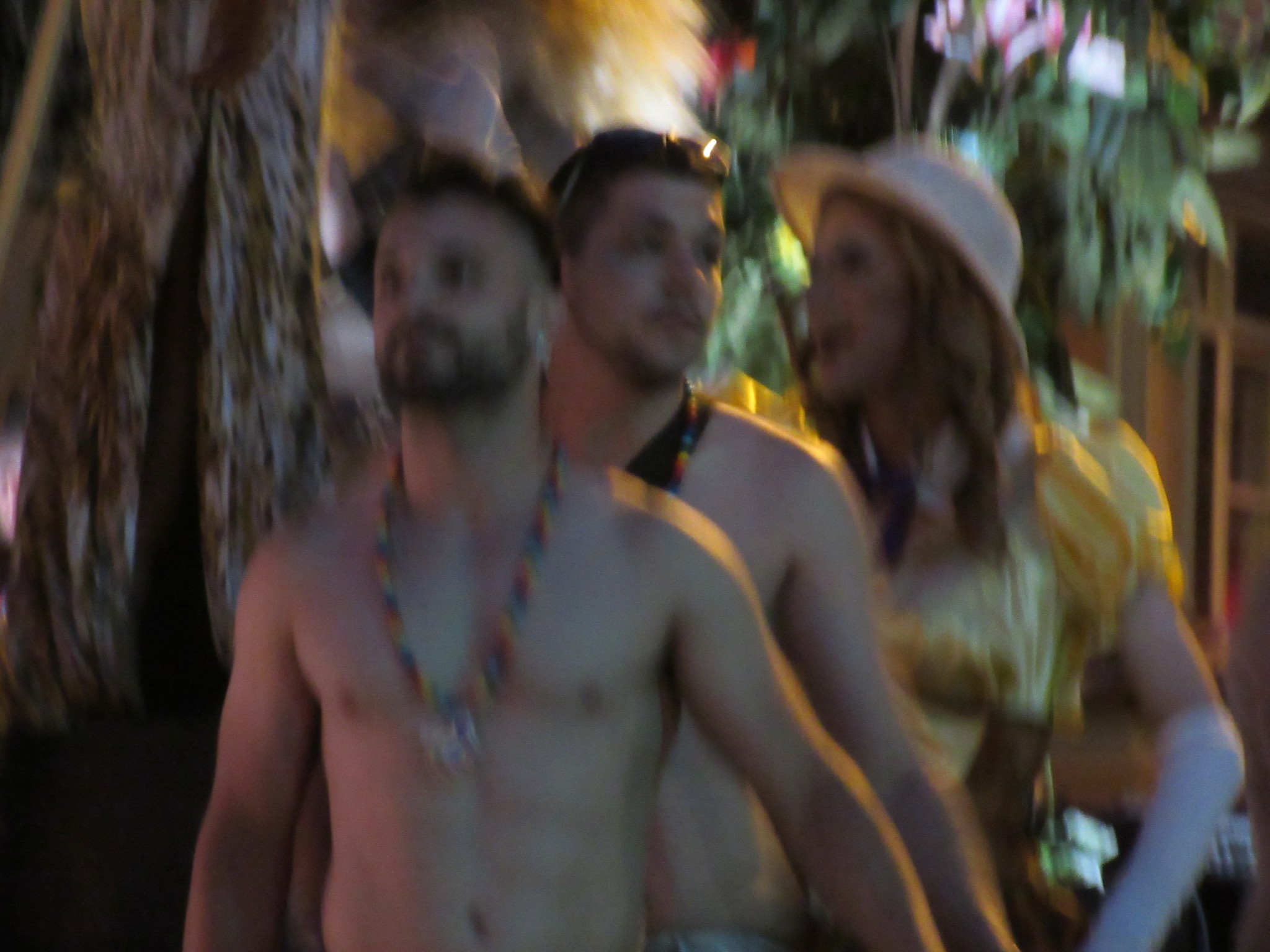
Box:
[534,369,700,500]
[368,433,571,777]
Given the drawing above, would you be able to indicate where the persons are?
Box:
[677,119,1250,952]
[283,122,1026,952]
[178,136,947,952]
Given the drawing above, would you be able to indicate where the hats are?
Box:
[773,135,1033,375]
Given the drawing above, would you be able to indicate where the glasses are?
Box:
[546,134,734,221]
